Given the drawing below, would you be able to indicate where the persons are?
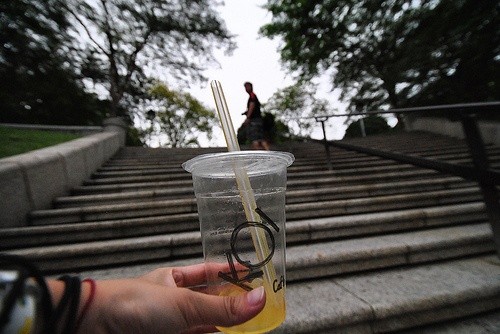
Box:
[241,81,270,150]
[0,254,265,334]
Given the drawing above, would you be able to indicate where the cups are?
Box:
[181,149,295,334]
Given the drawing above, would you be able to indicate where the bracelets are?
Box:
[52,273,96,334]
